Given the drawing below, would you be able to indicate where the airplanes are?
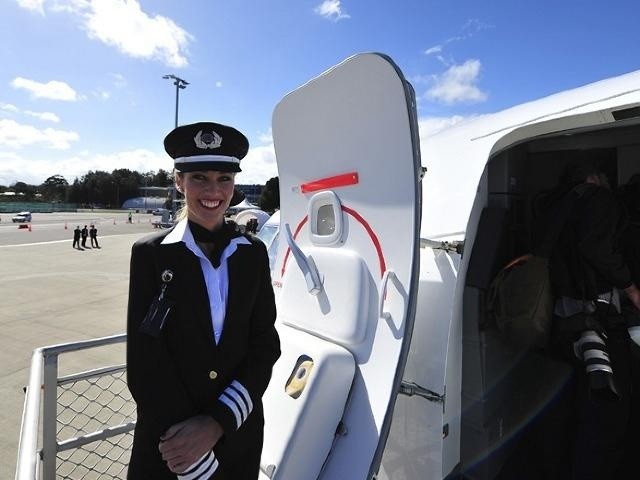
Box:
[120,196,184,216]
[252,51,639,479]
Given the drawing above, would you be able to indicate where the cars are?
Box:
[12,211,31,222]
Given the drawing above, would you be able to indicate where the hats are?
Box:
[165,122,251,173]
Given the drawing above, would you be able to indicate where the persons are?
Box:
[483,165,640,419]
[73,226,83,250]
[128,212,132,224]
[89,224,100,248]
[82,225,88,248]
[125,122,282,479]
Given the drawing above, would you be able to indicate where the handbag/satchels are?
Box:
[490,256,552,350]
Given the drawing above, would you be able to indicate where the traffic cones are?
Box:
[64,223,67,230]
[29,224,32,232]
[113,219,116,225]
